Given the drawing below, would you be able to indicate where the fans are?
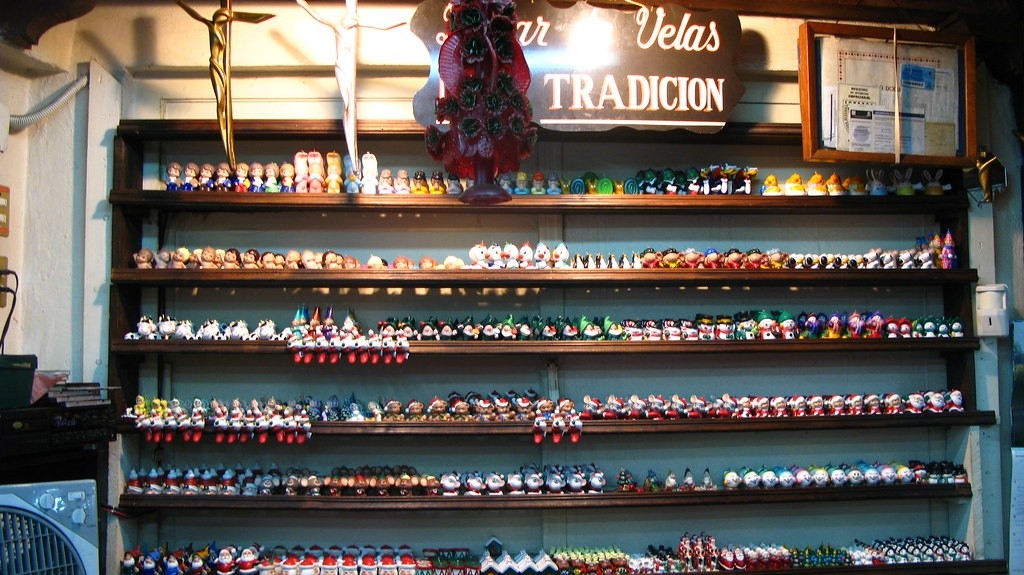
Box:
[0,479,99,575]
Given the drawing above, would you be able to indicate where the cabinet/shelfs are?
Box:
[106,119,1014,575]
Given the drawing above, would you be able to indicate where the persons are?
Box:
[120,539,415,575]
[919,228,957,269]
[133,393,312,444]
[133,159,559,271]
[385,387,965,444]
[287,305,409,365]
[679,532,790,573]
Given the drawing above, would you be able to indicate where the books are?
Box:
[49,382,121,406]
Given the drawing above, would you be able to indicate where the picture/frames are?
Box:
[797,21,978,169]
